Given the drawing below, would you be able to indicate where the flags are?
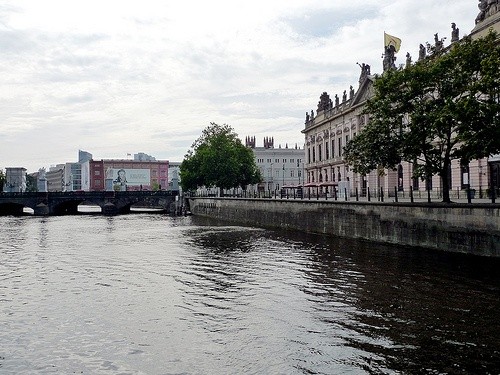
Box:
[384,33,402,53]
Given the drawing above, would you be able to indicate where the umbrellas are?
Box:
[282,180,338,188]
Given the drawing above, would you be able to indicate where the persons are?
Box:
[356,62,371,81]
[113,168,127,183]
[305,84,355,125]
[383,44,397,71]
[406,22,459,65]
[474,0,499,25]
